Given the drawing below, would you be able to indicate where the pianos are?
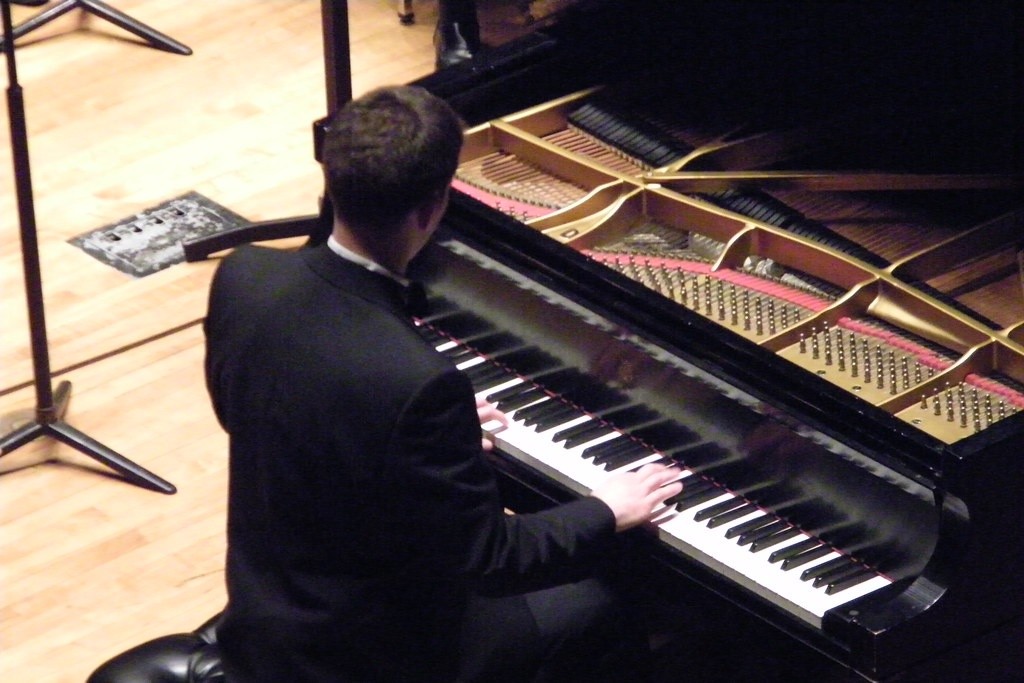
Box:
[303,0,1024,682]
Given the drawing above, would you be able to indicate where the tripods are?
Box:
[0,0,193,494]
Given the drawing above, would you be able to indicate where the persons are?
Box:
[203,86,683,683]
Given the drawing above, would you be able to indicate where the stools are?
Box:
[84,608,229,683]
